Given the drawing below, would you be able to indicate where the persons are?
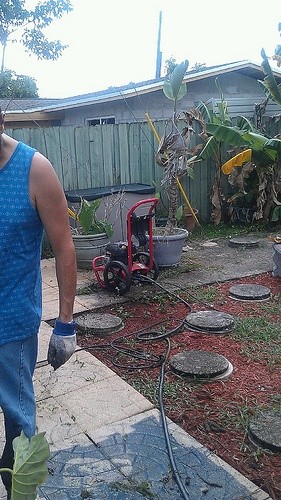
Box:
[0,106,77,500]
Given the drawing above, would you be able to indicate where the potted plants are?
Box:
[68,197,114,271]
[145,59,207,267]
[181,207,199,231]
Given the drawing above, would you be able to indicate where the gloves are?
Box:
[47,318,77,371]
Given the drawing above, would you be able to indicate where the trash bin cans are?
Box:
[64,183,156,241]
